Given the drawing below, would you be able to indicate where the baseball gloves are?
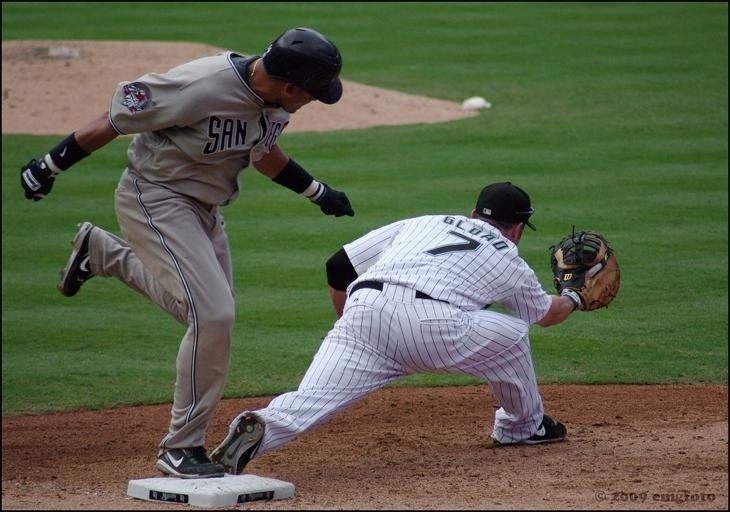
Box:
[548,225,620,311]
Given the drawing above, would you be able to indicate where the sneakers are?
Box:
[156,411,265,478]
[522,414,567,444]
[58,222,94,297]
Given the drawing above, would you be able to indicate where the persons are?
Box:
[19,28,353,476]
[208,181,620,475]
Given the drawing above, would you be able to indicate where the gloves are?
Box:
[301,179,354,217]
[20,153,62,202]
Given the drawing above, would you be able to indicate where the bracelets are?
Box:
[273,159,319,196]
[42,133,89,173]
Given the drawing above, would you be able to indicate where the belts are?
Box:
[349,282,450,304]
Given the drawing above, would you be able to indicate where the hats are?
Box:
[476,182,536,231]
[262,27,343,104]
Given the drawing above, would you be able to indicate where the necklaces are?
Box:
[247,56,265,83]
